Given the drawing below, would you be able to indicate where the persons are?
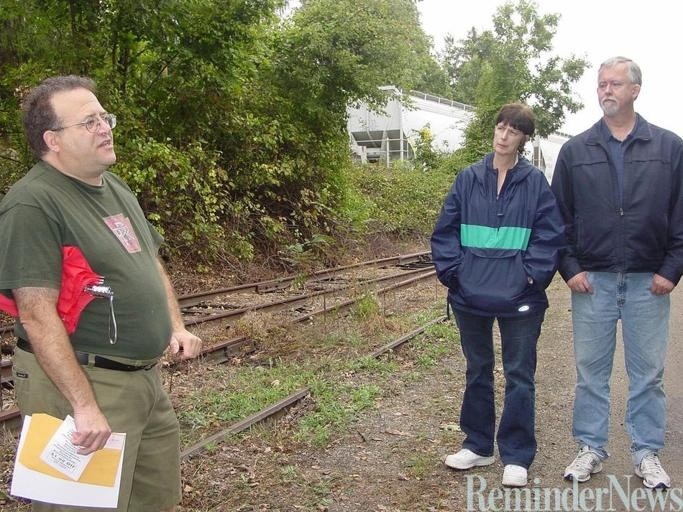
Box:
[431,103,565,486]
[0,76,202,512]
[550,56,683,491]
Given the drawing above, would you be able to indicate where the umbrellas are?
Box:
[0,246,111,336]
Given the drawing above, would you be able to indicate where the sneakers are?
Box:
[444,449,496,470]
[634,452,671,489]
[564,444,604,482]
[501,464,528,487]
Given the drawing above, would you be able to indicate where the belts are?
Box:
[16,334,158,372]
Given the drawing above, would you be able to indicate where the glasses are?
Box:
[48,112,117,133]
